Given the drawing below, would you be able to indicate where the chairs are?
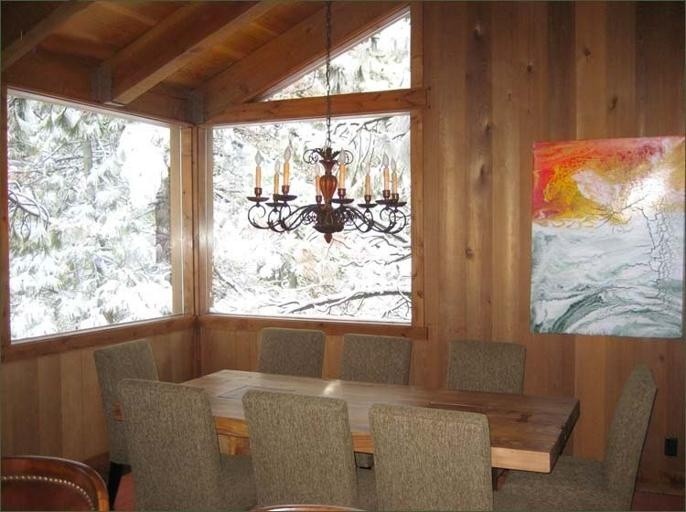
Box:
[243,389,375,511]
[445,340,527,478]
[118,379,254,511]
[367,403,526,512]
[1,456,109,512]
[258,326,325,379]
[501,363,658,512]
[339,333,414,470]
[95,339,161,512]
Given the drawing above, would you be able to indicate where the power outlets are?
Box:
[664,435,678,455]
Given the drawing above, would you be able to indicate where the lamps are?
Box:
[247,0,407,242]
